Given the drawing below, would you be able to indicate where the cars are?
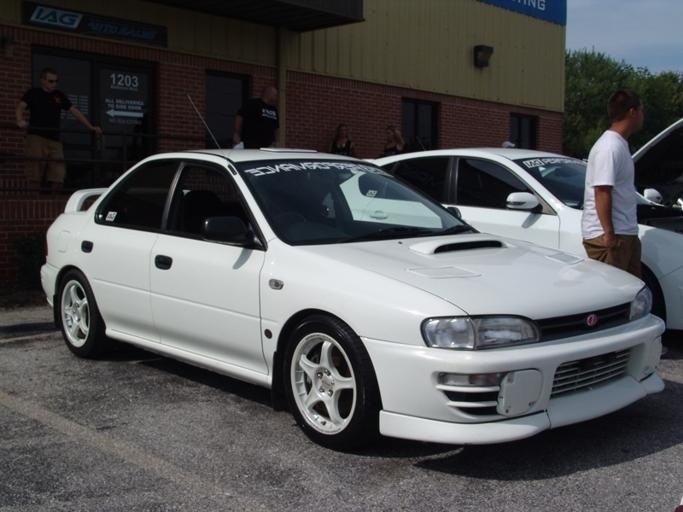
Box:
[40,146,666,454]
[327,116,683,333]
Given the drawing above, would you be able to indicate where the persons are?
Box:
[233,86,279,148]
[581,89,644,282]
[384,126,406,157]
[14,67,103,200]
[328,123,358,156]
[502,141,516,148]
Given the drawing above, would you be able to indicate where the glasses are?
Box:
[46,78,59,83]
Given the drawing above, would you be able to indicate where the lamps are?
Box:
[472,42,493,69]
